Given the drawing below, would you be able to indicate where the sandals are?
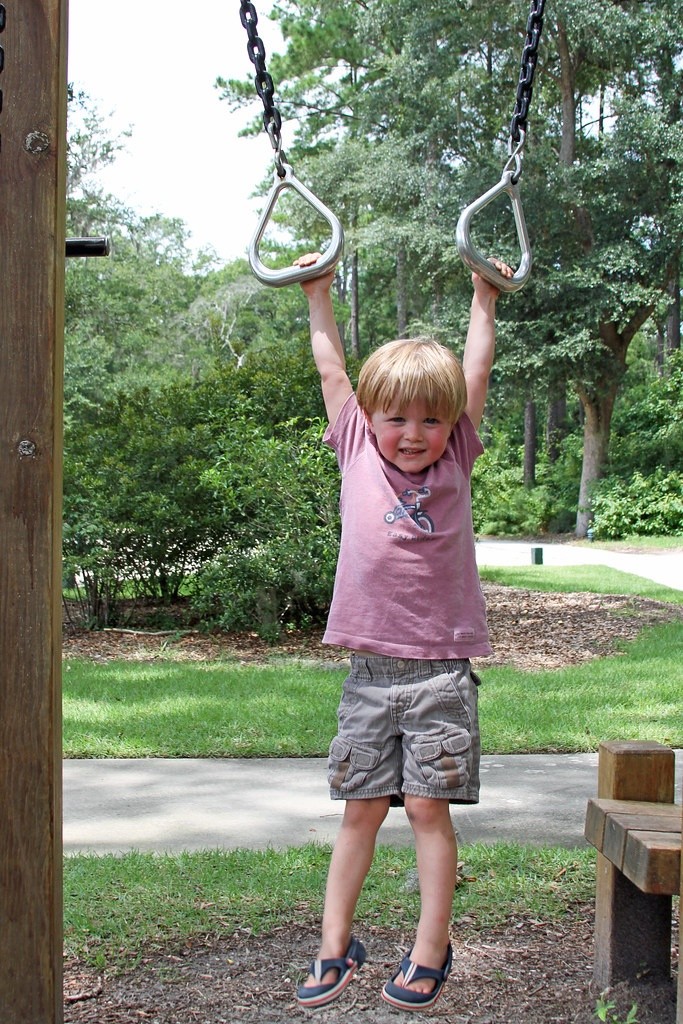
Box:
[381,940,453,1011]
[297,936,366,1007]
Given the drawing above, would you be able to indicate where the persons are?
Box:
[289,252,514,1011]
